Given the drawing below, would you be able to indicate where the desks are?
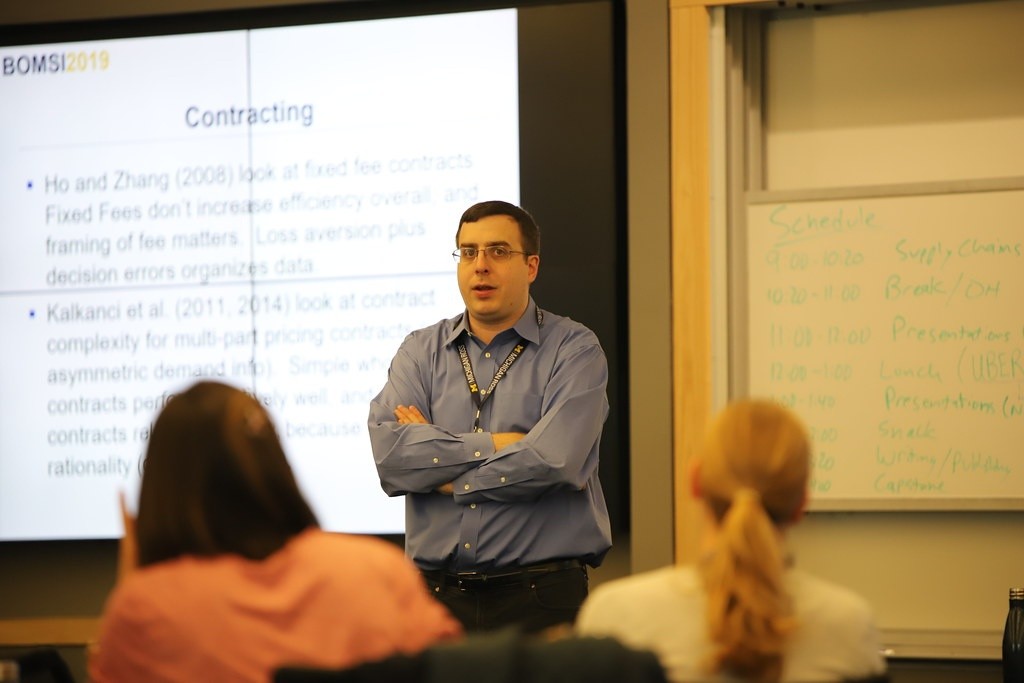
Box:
[0,614,101,681]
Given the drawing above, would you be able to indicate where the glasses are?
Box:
[451,246,535,264]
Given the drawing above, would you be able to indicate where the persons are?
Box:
[576,398,885,683]
[367,200,610,638]
[88,379,469,683]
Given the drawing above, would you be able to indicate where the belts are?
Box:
[418,558,584,593]
